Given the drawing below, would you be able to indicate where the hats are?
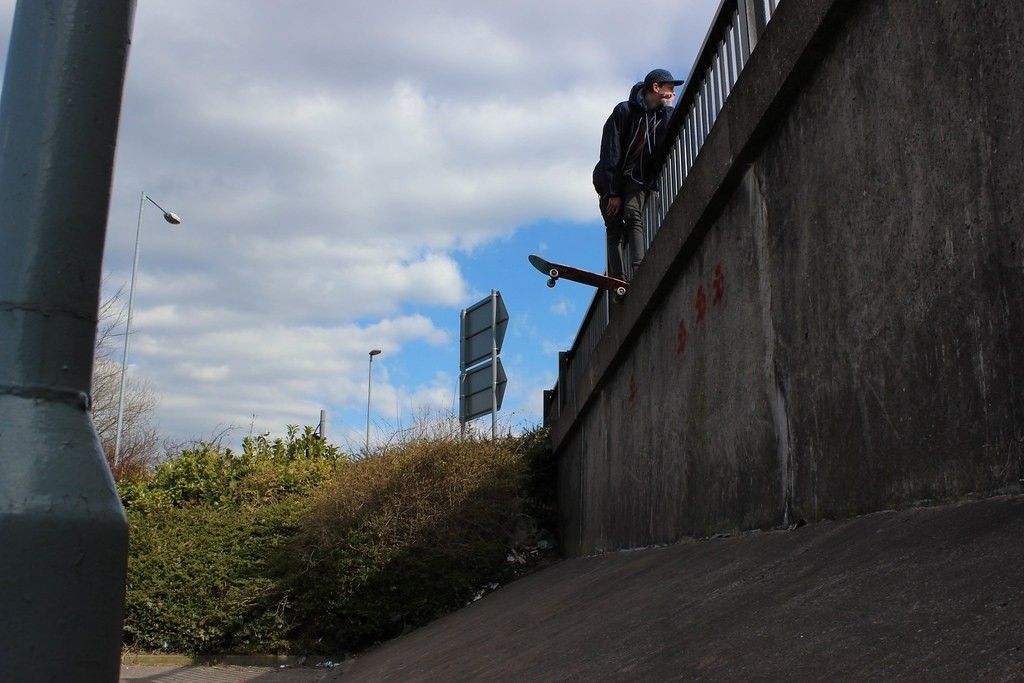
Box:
[644,69,684,89]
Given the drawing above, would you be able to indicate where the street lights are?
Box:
[365,349,383,452]
[111,190,183,468]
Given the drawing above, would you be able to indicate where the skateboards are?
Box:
[528,254,630,304]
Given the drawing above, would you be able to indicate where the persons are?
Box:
[590,68,687,290]
